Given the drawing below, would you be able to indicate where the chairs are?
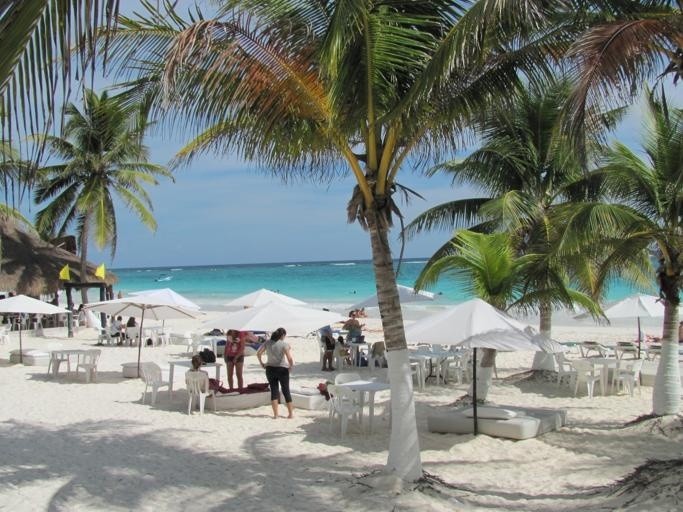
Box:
[319,337,498,444]
[99,324,169,347]
[142,359,223,415]
[187,333,226,358]
[47,349,101,383]
[554,341,661,398]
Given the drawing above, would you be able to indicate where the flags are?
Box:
[94,263,105,280]
[58,263,70,281]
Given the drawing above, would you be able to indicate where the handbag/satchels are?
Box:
[199,348,216,363]
[208,378,223,393]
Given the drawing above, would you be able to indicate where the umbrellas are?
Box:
[351,286,441,307]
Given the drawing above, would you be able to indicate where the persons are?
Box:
[343,311,365,352]
[256,327,293,418]
[224,329,245,390]
[184,355,217,398]
